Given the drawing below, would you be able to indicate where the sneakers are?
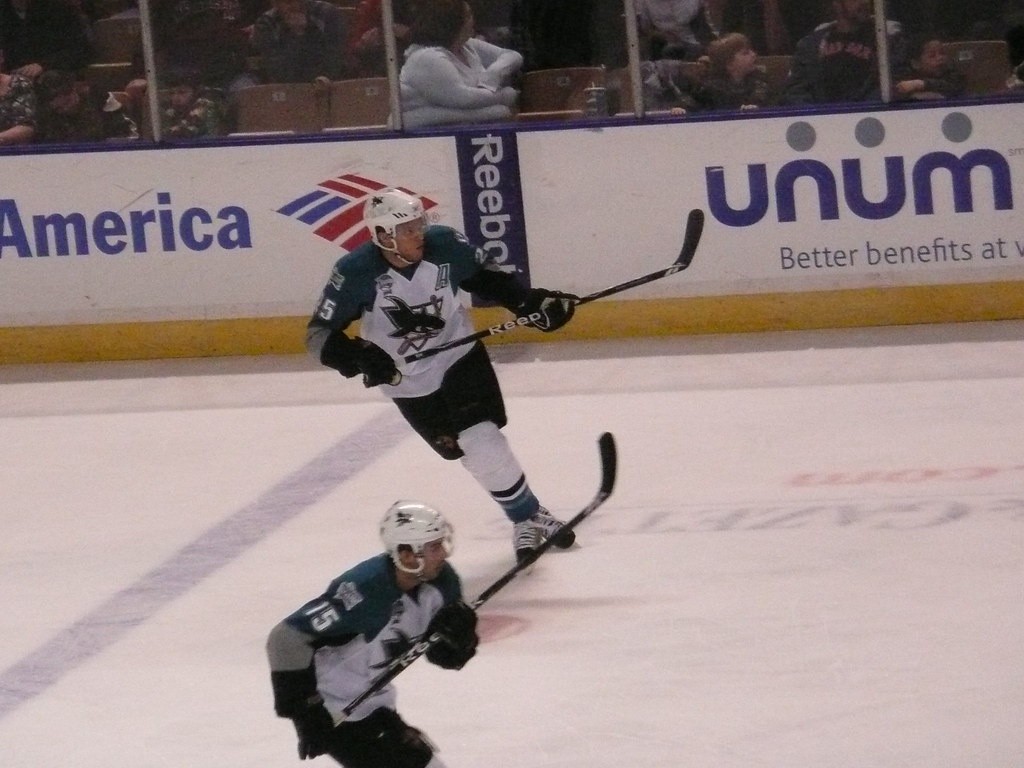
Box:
[532,504,581,550]
[514,519,539,564]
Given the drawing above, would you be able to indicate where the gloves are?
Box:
[423,600,477,648]
[283,693,334,759]
[318,330,395,388]
[497,281,580,333]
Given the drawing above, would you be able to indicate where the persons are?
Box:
[617,0,1024,115]
[302,187,575,573]
[0,0,441,147]
[266,501,479,768]
[387,0,523,127]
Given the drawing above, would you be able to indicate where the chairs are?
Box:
[77,13,1021,141]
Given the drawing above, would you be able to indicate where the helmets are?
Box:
[380,500,450,572]
[363,186,439,251]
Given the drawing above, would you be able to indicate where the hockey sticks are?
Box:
[362,208,706,388]
[331,432,623,728]
